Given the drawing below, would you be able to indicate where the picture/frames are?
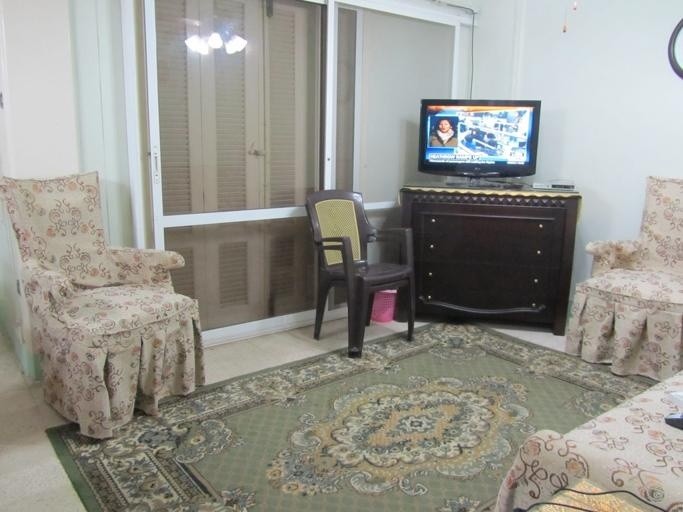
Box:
[668,18,683,79]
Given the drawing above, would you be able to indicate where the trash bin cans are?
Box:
[371,289,398,322]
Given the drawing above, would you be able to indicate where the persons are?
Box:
[431,118,457,147]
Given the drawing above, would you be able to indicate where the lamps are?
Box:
[184,23,248,56]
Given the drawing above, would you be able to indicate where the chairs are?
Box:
[306,190,416,358]
[496,369,683,512]
[0,170,204,440]
[565,176,683,379]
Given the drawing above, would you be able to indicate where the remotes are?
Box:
[551,184,574,188]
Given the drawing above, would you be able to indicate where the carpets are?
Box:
[45,323,661,512]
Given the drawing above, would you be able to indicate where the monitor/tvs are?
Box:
[418,100,542,189]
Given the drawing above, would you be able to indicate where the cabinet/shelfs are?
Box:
[401,186,582,335]
